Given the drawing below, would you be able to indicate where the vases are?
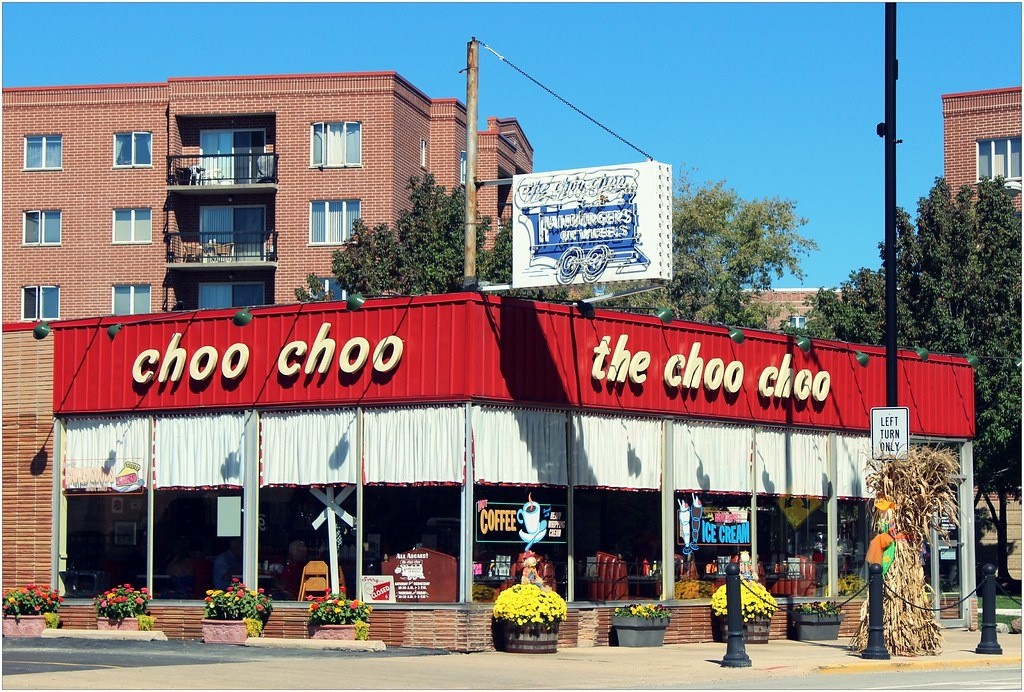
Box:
[202,619,247,643]
[612,617,669,647]
[2,615,47,638]
[792,614,845,640]
[97,618,139,631]
[719,612,771,643]
[308,624,356,640]
[502,620,559,653]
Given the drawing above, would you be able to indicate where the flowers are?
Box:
[796,601,842,616]
[307,587,373,641]
[205,578,273,637]
[2,583,64,628]
[710,579,778,622]
[94,585,154,630]
[614,603,672,619]
[492,583,566,631]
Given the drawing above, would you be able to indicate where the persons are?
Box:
[549,532,662,600]
[123,535,356,600]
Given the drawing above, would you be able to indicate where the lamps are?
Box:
[503,296,595,320]
[719,322,744,343]
[107,309,206,338]
[347,294,407,311]
[33,313,115,339]
[595,306,672,323]
[779,331,811,351]
[232,301,310,326]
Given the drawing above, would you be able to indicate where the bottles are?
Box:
[642,559,657,576]
[711,559,718,574]
[490,559,495,578]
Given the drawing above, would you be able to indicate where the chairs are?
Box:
[182,242,234,261]
[189,166,221,185]
